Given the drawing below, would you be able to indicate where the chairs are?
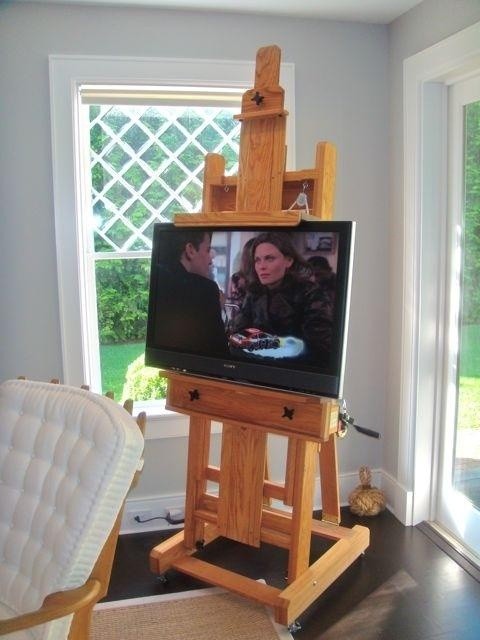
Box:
[0,374,148,638]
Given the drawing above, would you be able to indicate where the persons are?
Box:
[226,232,334,360]
[303,255,339,323]
[150,230,261,366]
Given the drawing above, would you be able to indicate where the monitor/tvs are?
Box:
[145,221,356,400]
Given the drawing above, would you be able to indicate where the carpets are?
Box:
[89,577,294,640]
